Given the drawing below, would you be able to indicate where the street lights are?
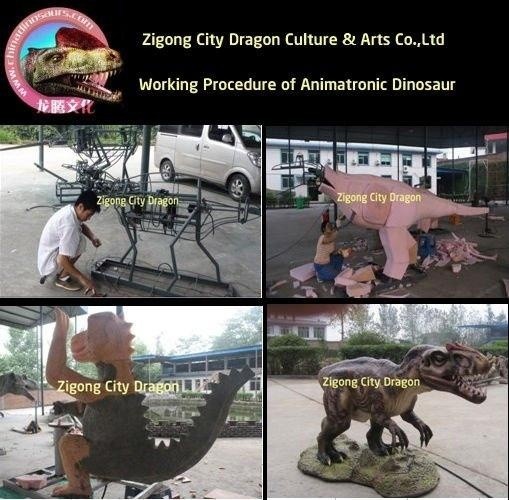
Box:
[471,147,489,196]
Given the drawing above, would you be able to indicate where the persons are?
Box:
[313,221,352,283]
[37,190,102,296]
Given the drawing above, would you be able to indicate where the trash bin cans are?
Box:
[366,228,383,250]
[297,197,305,209]
[48,414,83,476]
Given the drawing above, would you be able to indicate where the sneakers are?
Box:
[316,270,325,283]
[54,272,82,290]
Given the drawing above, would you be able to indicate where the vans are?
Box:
[154,125,261,203]
